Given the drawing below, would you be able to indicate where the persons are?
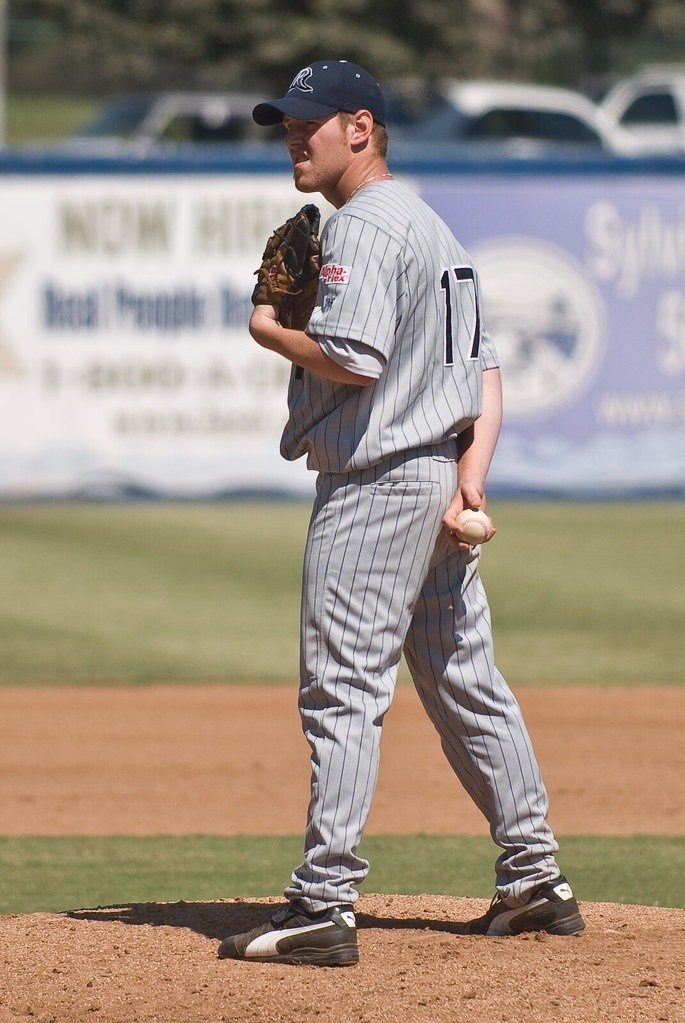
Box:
[216,59,586,968]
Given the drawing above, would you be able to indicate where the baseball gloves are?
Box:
[250,202,323,335]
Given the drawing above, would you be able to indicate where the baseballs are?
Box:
[456,508,492,545]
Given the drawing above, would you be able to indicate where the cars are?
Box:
[432,67,685,158]
[71,88,283,144]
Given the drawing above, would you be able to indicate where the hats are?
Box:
[252,60,387,130]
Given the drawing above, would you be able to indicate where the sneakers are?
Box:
[218,900,359,966]
[463,875,586,936]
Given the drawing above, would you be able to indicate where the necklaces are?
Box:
[347,173,392,204]
